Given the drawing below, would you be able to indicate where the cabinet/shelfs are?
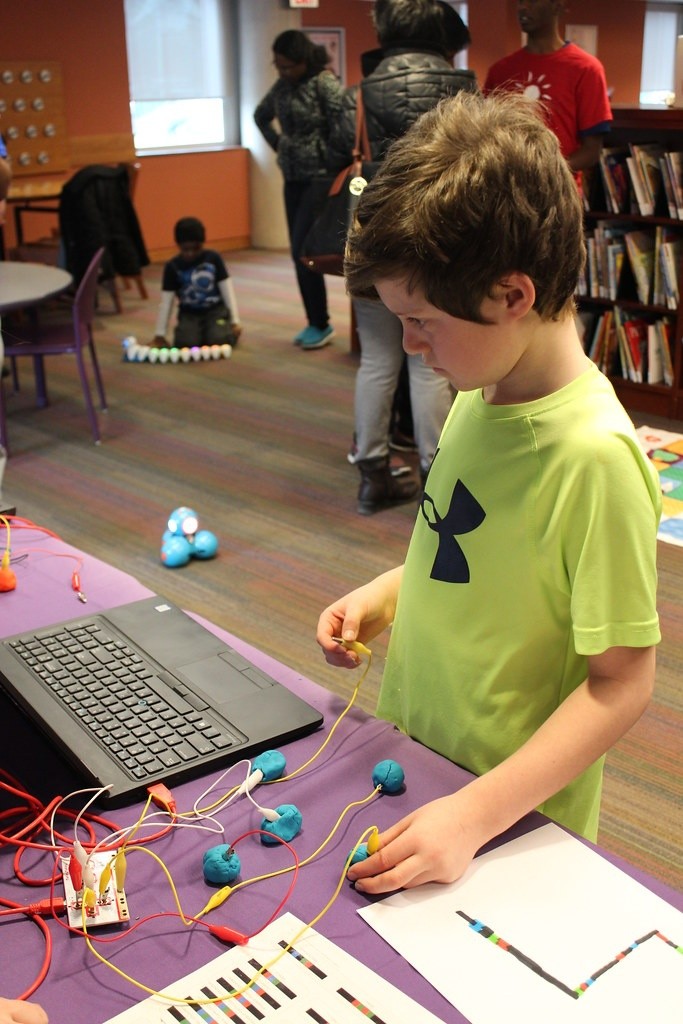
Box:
[570,108,683,423]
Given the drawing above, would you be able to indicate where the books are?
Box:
[572,139,683,387]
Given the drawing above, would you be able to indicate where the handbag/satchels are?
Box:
[299,87,384,275]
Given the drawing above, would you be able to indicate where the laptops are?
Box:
[0,593,324,810]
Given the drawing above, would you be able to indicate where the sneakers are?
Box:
[388,433,417,451]
[347,433,412,477]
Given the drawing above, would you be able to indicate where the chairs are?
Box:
[0,244,108,448]
[10,160,148,314]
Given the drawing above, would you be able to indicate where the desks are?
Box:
[5,168,78,246]
[0,260,74,306]
[0,518,683,1024]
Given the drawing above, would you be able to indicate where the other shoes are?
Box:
[295,324,335,348]
[358,454,419,515]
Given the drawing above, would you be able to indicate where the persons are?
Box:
[343,1,476,517]
[316,93,663,897]
[482,0,614,170]
[254,30,345,350]
[142,218,242,348]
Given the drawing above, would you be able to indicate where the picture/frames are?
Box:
[301,26,347,87]
